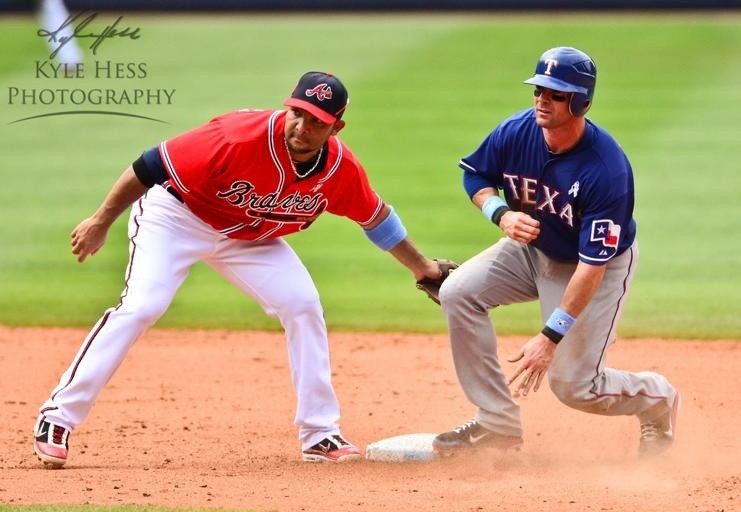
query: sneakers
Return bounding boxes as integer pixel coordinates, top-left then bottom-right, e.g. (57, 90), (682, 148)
(432, 418), (524, 456)
(33, 414), (70, 468)
(633, 389), (682, 466)
(302, 433), (363, 465)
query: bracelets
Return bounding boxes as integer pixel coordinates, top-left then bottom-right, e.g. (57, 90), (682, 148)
(542, 308), (576, 344)
(481, 195), (509, 224)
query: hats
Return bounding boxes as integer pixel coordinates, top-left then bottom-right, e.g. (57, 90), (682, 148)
(283, 70), (350, 126)
(522, 46), (597, 118)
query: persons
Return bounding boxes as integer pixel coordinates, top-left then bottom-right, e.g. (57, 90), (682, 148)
(433, 46), (679, 461)
(34, 71), (459, 464)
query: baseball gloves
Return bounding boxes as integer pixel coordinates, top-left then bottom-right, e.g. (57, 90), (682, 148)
(419, 259), (460, 303)
